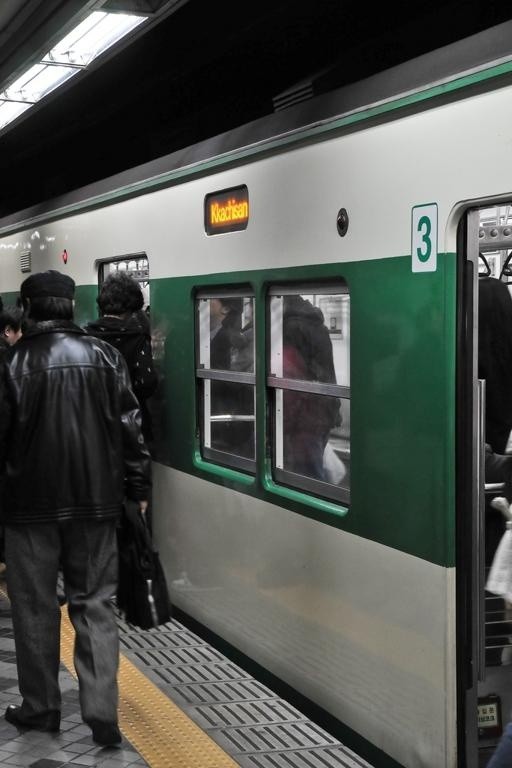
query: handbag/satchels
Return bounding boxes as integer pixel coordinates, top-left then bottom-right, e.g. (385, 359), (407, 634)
(117, 513), (170, 629)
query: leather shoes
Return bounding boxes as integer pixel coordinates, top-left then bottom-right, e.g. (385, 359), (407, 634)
(93, 725), (121, 744)
(6, 704), (61, 731)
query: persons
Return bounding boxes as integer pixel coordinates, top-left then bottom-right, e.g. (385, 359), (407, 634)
(0, 270), (152, 743)
(282, 294), (343, 483)
(2, 305), (22, 360)
(209, 297), (255, 460)
(81, 270), (155, 446)
(483, 443), (512, 666)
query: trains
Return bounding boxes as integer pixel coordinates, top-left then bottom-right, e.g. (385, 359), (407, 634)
(2, 15), (511, 768)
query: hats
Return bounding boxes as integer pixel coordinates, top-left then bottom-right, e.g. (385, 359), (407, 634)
(21, 270), (75, 298)
